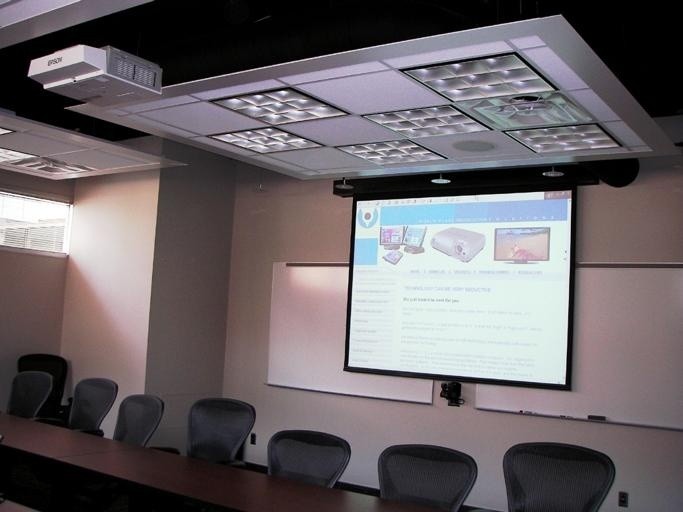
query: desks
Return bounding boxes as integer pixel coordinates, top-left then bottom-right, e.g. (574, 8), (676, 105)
(0, 410), (448, 512)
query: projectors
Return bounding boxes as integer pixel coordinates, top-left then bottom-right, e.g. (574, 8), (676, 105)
(28, 44), (163, 108)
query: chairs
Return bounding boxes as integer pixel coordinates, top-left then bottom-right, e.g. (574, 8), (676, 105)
(142, 394), (258, 471)
(264, 427), (352, 491)
(500, 440), (618, 511)
(14, 351), (69, 408)
(6, 370), (54, 424)
(30, 375), (118, 440)
(108, 391), (166, 447)
(375, 441), (479, 512)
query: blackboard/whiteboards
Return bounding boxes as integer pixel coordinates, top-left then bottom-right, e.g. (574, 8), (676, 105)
(263, 260), (434, 406)
(473, 261), (683, 432)
